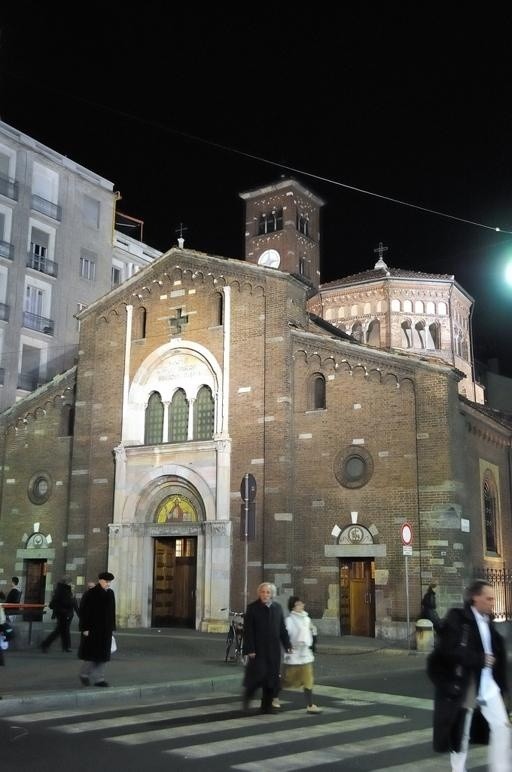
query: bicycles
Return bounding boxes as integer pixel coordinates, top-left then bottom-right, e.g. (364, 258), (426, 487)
(217, 606), (249, 665)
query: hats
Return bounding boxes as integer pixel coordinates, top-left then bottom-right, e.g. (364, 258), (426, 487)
(98, 572), (114, 581)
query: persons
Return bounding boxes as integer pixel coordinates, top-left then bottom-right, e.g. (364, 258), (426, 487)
(5, 577), (22, 626)
(0, 591), (11, 666)
(272, 595), (323, 713)
(66, 583), (80, 648)
(421, 582), (440, 632)
(79, 572), (116, 688)
(432, 579), (511, 771)
(38, 575), (75, 653)
(239, 582), (292, 715)
(77, 581), (96, 660)
(165, 499), (183, 522)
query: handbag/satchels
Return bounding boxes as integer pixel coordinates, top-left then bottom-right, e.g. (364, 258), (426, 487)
(111, 637), (118, 653)
(426, 647), (468, 699)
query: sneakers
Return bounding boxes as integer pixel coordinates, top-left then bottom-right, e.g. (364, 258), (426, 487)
(80, 676), (108, 687)
(262, 697), (323, 715)
